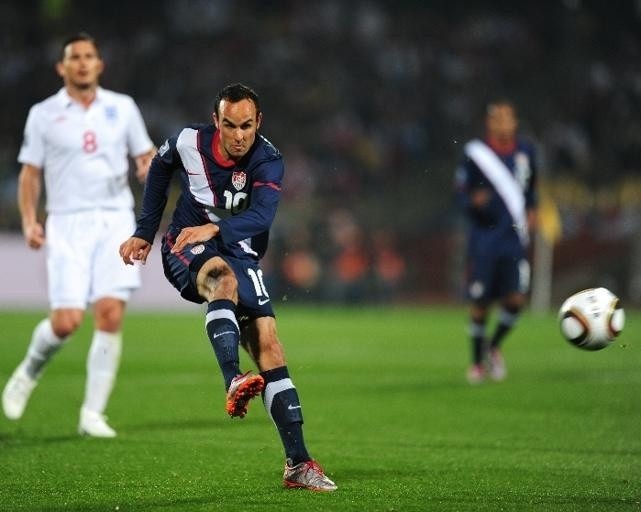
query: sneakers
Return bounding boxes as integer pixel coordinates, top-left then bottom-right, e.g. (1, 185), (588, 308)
(1, 359), (39, 422)
(281, 455), (339, 493)
(78, 403), (117, 439)
(462, 346), (506, 387)
(223, 369), (266, 422)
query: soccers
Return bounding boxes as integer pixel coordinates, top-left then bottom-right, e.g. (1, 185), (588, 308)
(558, 285), (627, 351)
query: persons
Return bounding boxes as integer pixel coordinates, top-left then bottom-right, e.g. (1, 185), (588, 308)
(450, 95), (540, 387)
(0, 32), (161, 440)
(117, 83), (341, 492)
